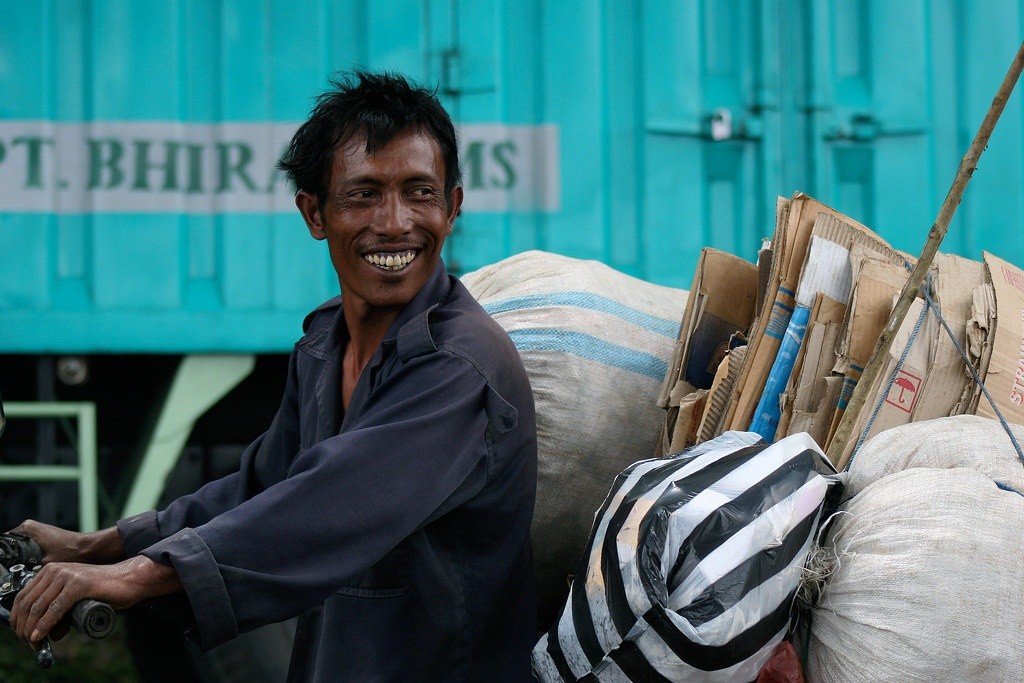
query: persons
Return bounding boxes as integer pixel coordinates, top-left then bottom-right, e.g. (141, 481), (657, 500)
(5, 68), (540, 683)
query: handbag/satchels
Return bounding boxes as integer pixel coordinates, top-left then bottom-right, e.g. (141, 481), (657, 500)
(527, 429), (842, 683)
